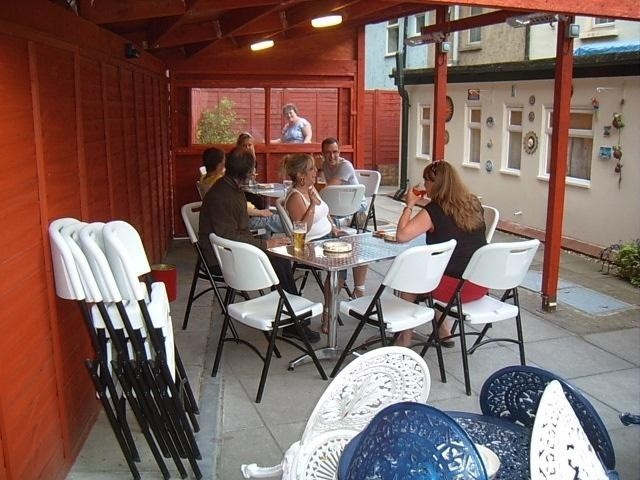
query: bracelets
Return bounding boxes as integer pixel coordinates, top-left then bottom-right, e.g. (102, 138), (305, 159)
(307, 208), (315, 215)
(330, 223), (337, 228)
(403, 205), (413, 213)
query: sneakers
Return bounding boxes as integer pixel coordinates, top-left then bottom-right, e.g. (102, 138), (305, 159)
(349, 289), (366, 301)
(282, 324), (321, 344)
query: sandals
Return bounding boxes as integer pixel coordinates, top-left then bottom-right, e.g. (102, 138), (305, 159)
(322, 308), (330, 334)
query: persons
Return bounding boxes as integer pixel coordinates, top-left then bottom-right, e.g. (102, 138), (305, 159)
(283, 154), (367, 333)
(391, 160), (488, 349)
(224, 130), (266, 207)
(308, 138), (368, 229)
(196, 147), (319, 343)
(263, 102), (313, 144)
(196, 146), (290, 234)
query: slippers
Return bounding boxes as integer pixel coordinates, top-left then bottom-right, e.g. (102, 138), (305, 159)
(432, 335), (455, 349)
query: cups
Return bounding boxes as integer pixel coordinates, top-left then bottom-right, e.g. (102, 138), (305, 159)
(316, 176), (326, 190)
(292, 219), (307, 250)
(282, 175), (294, 196)
(412, 182), (426, 196)
(248, 177), (257, 187)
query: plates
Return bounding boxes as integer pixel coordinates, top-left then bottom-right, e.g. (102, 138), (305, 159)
(322, 241), (353, 253)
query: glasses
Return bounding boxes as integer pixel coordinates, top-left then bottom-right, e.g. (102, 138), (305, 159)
(430, 161), (439, 176)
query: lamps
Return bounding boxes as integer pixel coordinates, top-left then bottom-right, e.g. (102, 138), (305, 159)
(310, 14), (343, 29)
(250, 40), (274, 52)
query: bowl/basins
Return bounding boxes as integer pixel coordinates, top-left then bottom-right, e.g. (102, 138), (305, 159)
(433, 441), (501, 480)
